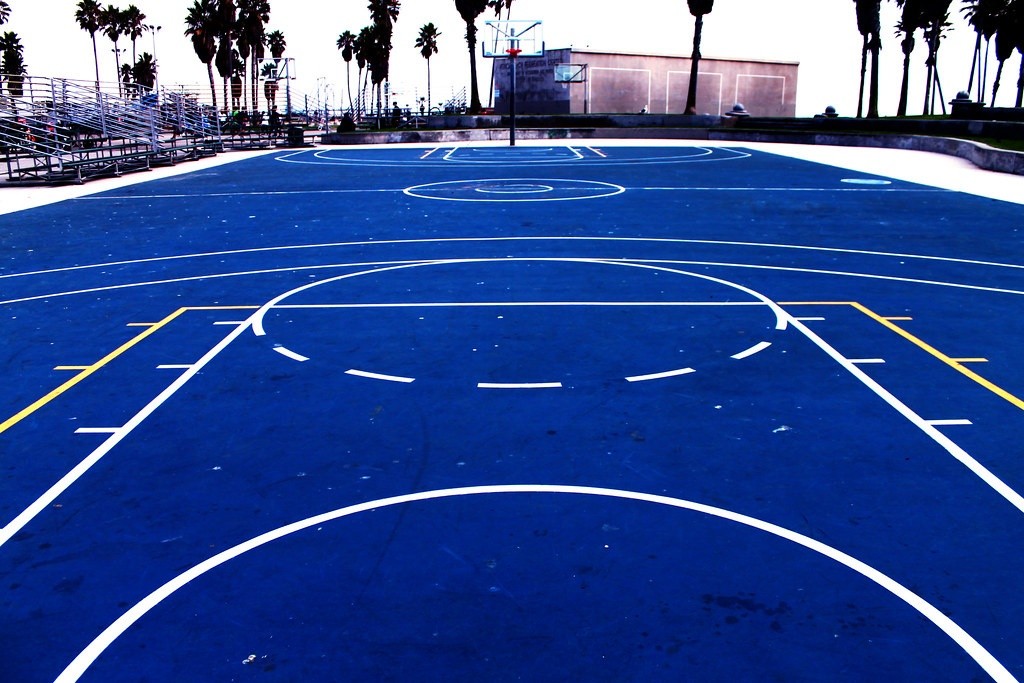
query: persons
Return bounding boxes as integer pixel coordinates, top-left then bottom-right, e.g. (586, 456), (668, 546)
(205, 106), (282, 137)
(392, 101), (400, 125)
